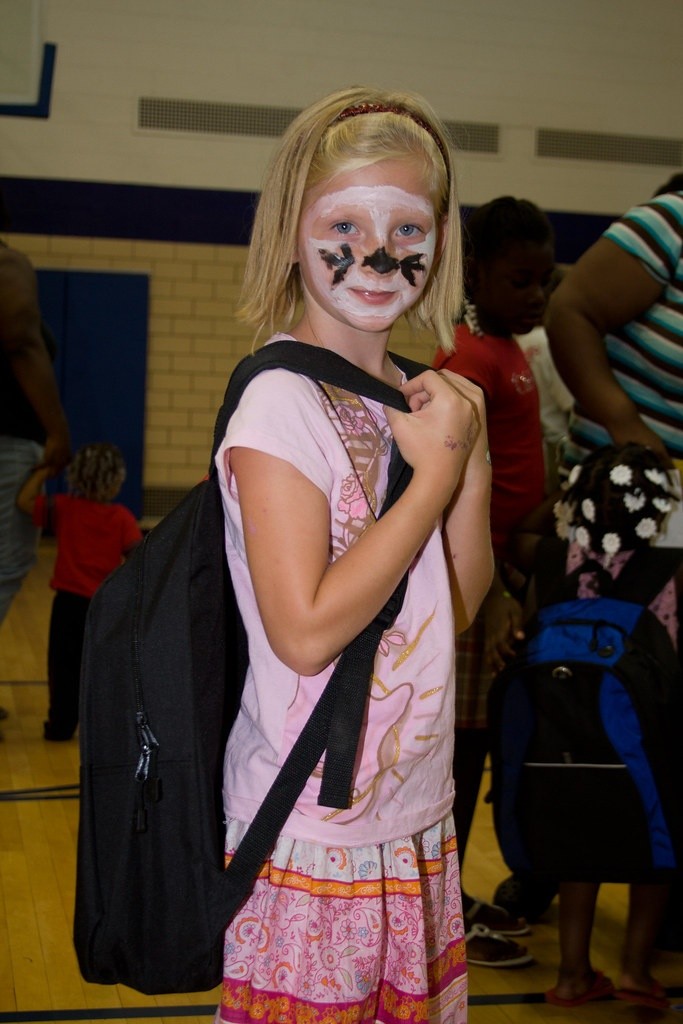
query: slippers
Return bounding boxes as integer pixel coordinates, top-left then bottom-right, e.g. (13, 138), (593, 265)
(465, 901), (530, 935)
(464, 923), (533, 966)
(545, 969), (614, 1007)
(614, 982), (669, 1008)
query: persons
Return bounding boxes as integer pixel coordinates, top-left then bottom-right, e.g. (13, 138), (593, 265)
(434, 161), (683, 1009)
(213, 86), (496, 1024)
(16, 431), (143, 744)
(0, 240), (76, 743)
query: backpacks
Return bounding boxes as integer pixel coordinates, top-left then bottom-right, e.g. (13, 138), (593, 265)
(484, 547), (683, 884)
(72, 340), (438, 995)
